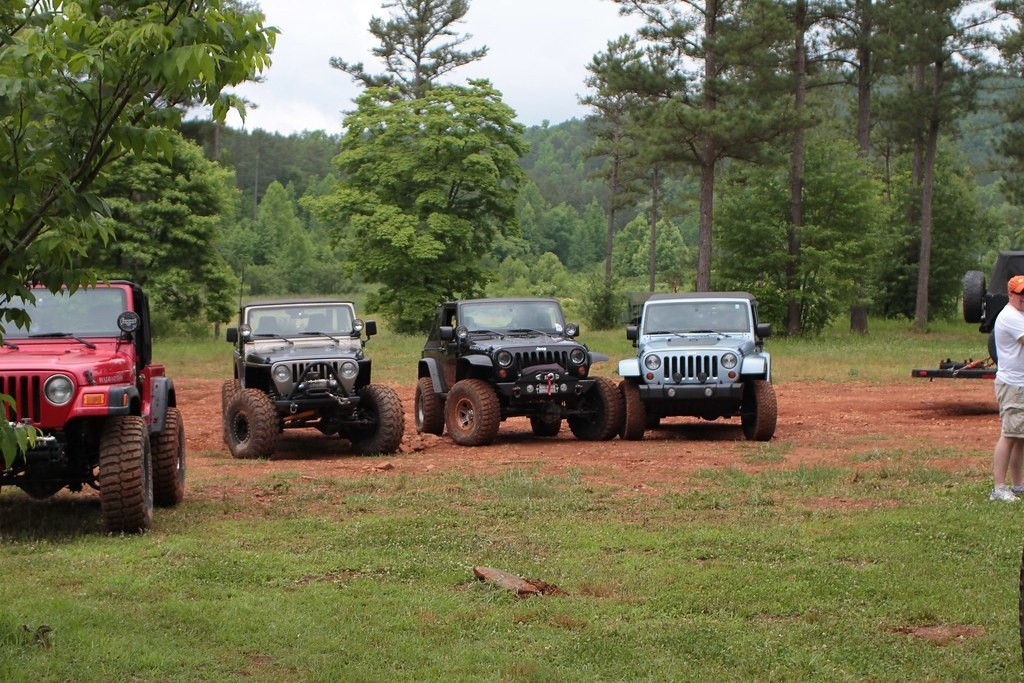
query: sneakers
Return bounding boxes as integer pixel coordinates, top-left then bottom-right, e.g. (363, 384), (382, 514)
(990, 483), (1024, 503)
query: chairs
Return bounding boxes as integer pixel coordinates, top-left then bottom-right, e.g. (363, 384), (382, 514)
(304, 313), (329, 331)
(253, 316), (282, 334)
(79, 302), (120, 331)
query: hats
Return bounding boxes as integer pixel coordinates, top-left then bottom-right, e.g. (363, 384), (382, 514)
(1008, 276), (1024, 296)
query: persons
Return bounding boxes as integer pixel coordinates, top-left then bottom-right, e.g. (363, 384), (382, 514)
(990, 276), (1024, 502)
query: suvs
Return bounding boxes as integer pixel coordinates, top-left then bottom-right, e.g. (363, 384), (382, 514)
(962, 251), (1024, 367)
(619, 290), (778, 442)
(221, 299), (406, 460)
(415, 298), (624, 446)
(0, 280), (185, 533)
